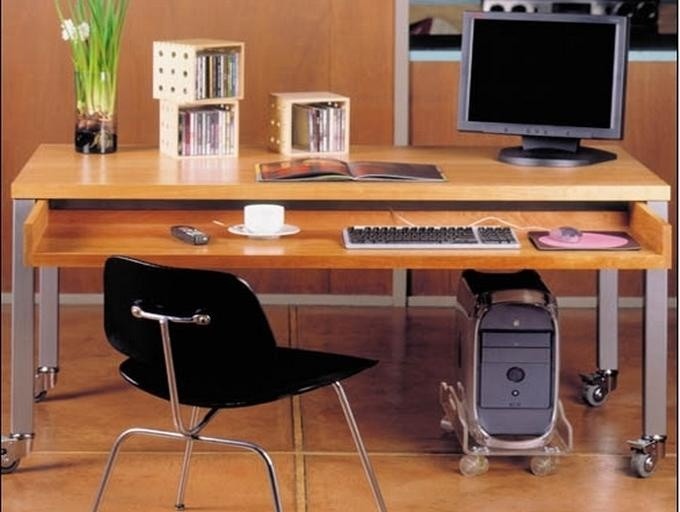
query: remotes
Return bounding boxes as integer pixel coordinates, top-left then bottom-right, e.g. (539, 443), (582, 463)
(171, 225), (210, 245)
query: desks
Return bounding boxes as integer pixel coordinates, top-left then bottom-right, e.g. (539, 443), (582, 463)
(1, 141), (673, 482)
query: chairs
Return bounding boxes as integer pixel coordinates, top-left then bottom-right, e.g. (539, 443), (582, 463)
(88, 253), (387, 512)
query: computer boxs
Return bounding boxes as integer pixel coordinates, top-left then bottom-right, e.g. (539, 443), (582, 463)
(451, 269), (560, 450)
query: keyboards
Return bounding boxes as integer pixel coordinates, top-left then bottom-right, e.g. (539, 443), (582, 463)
(343, 224), (522, 249)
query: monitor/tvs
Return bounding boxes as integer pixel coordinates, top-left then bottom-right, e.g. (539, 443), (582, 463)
(456, 9), (632, 167)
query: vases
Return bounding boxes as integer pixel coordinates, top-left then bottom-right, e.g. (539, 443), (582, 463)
(72, 69), (119, 154)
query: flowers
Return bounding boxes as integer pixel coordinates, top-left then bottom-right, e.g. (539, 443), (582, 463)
(56, 1), (128, 117)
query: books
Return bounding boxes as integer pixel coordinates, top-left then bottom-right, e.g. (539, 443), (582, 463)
(290, 103), (346, 154)
(253, 156), (449, 184)
(177, 51), (241, 156)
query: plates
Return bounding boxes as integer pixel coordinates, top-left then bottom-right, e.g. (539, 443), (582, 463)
(230, 223), (300, 239)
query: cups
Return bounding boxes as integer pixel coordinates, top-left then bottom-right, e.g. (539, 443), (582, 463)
(244, 204), (284, 232)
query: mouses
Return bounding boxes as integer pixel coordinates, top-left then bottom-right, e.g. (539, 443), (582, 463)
(549, 226), (581, 244)
